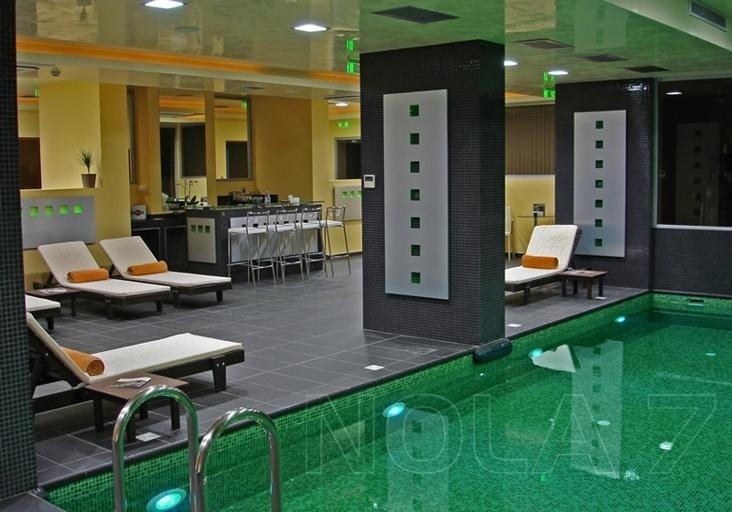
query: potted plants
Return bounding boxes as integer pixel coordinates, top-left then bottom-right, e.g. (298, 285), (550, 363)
(76, 145), (96, 188)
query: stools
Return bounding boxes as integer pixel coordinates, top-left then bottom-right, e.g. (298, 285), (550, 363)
(558, 270), (607, 298)
(25, 288), (79, 316)
(84, 370), (189, 442)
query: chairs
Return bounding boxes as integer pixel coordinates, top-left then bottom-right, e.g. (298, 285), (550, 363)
(227, 203), (352, 288)
(504, 225), (582, 304)
(26, 312), (245, 418)
(33, 241), (171, 320)
(25, 294), (60, 329)
(99, 236), (232, 309)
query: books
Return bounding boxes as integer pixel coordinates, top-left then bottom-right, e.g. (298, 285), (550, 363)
(108, 377), (151, 389)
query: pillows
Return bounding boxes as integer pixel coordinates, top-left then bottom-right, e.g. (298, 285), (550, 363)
(521, 254), (558, 269)
(67, 268), (108, 283)
(128, 260), (167, 275)
(63, 348), (104, 376)
